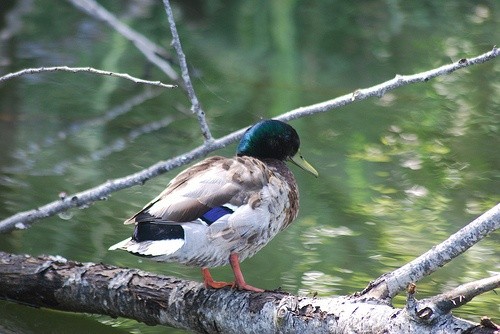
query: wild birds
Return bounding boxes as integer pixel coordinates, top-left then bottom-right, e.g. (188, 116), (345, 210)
(108, 119), (318, 293)
